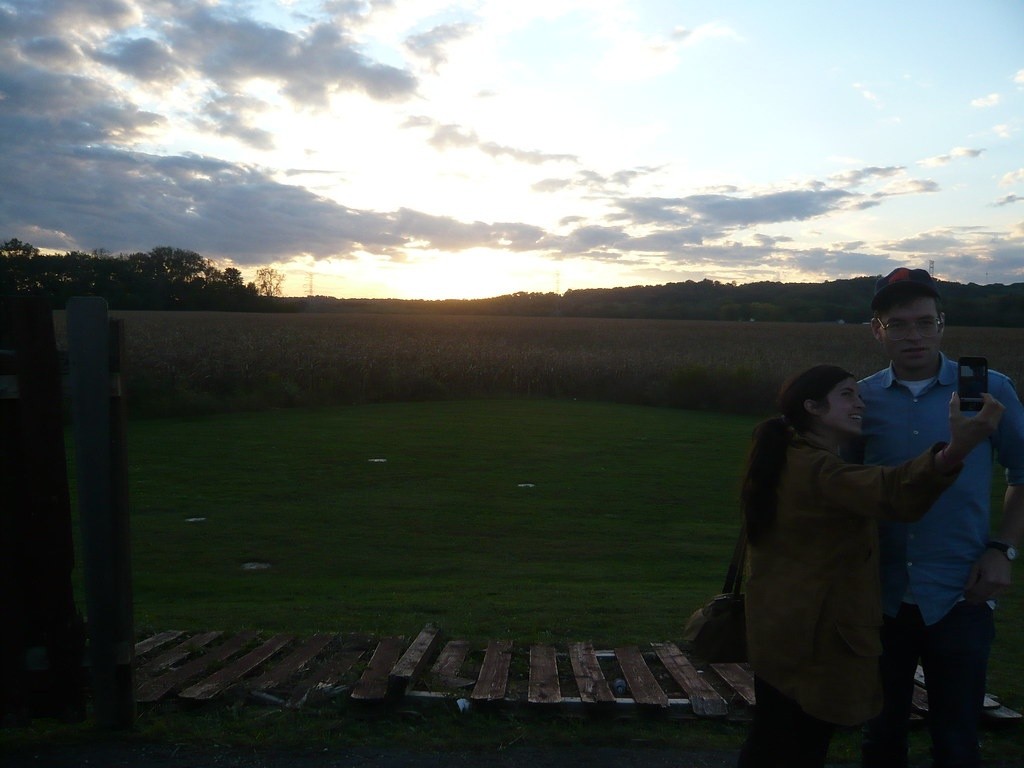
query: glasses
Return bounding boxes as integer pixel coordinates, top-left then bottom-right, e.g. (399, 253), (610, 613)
(877, 315), (943, 341)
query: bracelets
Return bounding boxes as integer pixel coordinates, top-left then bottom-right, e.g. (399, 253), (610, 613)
(942, 444), (950, 472)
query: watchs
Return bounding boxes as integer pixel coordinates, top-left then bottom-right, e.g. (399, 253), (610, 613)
(987, 540), (1017, 562)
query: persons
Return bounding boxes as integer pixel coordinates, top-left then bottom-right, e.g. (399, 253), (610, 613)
(851, 265), (1024, 767)
(737, 364), (1005, 767)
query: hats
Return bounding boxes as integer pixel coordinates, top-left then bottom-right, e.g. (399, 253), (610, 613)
(870, 266), (939, 312)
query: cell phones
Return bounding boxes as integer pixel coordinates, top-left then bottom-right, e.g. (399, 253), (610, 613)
(957, 356), (988, 412)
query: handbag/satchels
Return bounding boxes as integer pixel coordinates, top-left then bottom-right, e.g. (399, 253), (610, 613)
(684, 525), (751, 665)
